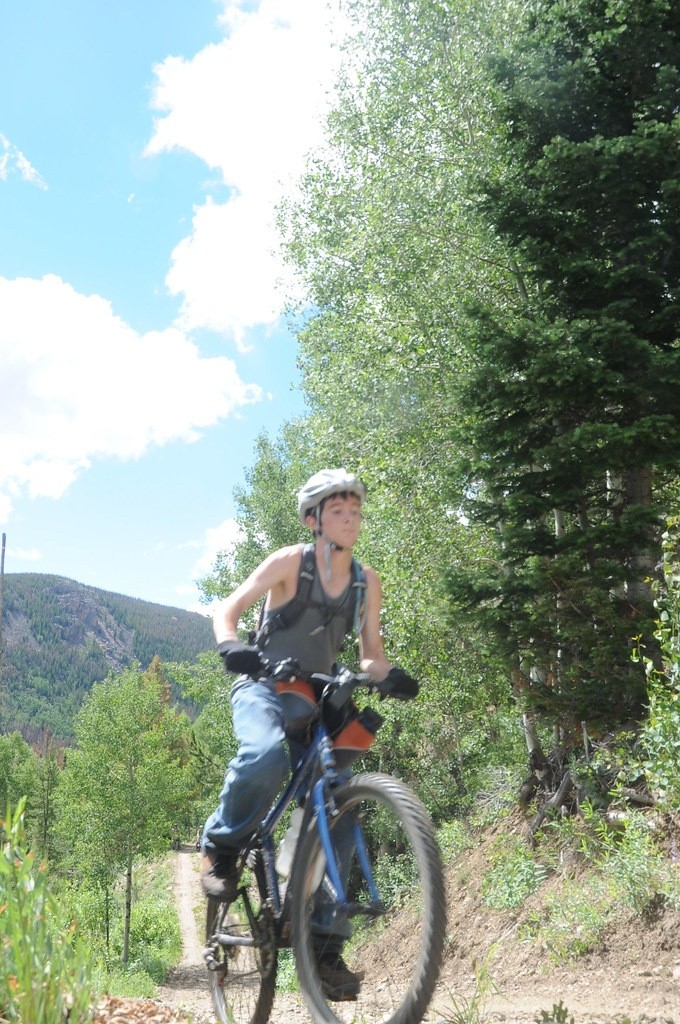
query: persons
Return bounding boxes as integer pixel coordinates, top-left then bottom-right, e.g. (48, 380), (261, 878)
(173, 824), (181, 847)
(196, 825), (204, 846)
(199, 467), (414, 1002)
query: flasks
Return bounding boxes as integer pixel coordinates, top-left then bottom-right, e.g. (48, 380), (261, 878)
(333, 707), (384, 752)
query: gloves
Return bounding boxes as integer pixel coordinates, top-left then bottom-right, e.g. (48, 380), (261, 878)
(376, 668), (419, 701)
(215, 641), (260, 675)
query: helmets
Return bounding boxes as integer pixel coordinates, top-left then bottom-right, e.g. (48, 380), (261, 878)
(297, 468), (366, 524)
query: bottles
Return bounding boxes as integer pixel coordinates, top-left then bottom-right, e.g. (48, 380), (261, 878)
(275, 797), (308, 878)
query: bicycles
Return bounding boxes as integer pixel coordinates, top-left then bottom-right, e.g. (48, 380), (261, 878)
(206, 651), (449, 1023)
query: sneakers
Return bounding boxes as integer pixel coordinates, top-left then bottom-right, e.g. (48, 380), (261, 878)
(314, 943), (362, 1003)
(199, 848), (239, 902)
